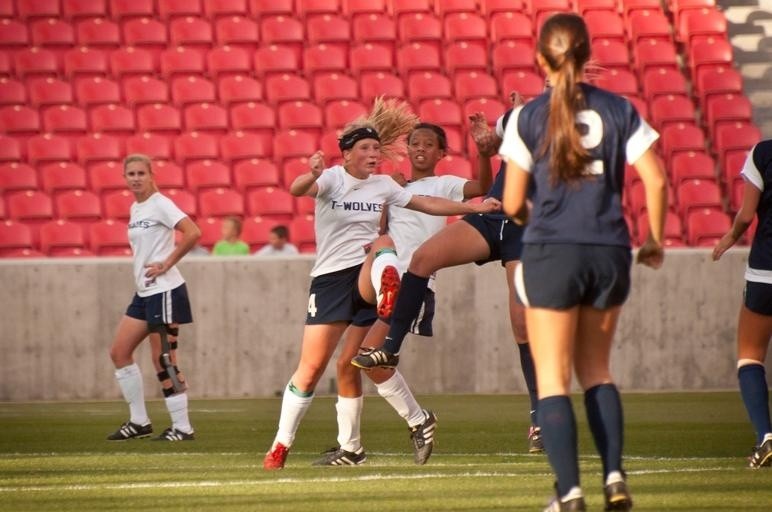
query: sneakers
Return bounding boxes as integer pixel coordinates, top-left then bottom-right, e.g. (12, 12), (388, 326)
(603, 482), (633, 512)
(377, 265), (401, 321)
(528, 426), (545, 453)
(350, 347), (400, 371)
(148, 427), (195, 442)
(310, 445), (368, 467)
(263, 439), (292, 470)
(408, 409), (438, 465)
(746, 440), (771, 468)
(106, 421), (152, 442)
(542, 495), (586, 512)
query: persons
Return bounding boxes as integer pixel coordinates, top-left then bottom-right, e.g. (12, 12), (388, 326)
(348, 56), (603, 458)
(174, 215), (209, 256)
(312, 108), (496, 467)
(250, 225), (300, 257)
(715, 139), (772, 469)
(263, 93), (504, 472)
(101, 153), (203, 444)
(496, 12), (670, 512)
(210, 218), (250, 257)
